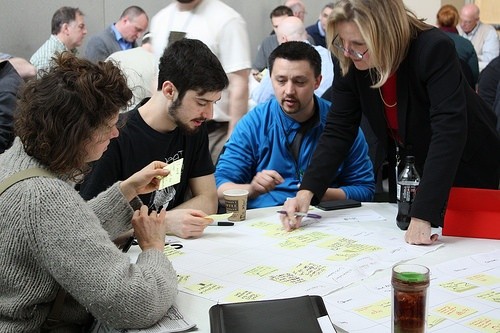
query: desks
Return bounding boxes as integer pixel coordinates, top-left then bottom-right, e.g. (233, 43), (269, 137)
(127, 202), (500, 333)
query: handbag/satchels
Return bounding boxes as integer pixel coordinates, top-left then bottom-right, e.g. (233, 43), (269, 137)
(209, 295), (337, 333)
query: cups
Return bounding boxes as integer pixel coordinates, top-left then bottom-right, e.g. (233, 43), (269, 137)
(391, 264), (431, 333)
(223, 189), (249, 222)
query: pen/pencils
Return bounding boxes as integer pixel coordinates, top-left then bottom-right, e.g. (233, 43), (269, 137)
(208, 221), (235, 226)
(277, 210), (322, 219)
(121, 203), (155, 256)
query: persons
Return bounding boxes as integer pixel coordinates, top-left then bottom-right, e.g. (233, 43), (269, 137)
(0, 0), (500, 333)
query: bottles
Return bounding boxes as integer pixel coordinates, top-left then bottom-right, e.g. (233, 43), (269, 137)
(396, 155), (421, 230)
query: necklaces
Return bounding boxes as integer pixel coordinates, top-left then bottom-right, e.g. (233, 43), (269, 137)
(377, 71), (397, 107)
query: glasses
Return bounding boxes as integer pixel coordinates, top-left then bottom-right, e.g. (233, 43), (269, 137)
(332, 34), (369, 59)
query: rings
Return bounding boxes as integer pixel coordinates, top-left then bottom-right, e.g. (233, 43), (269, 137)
(152, 212), (157, 217)
